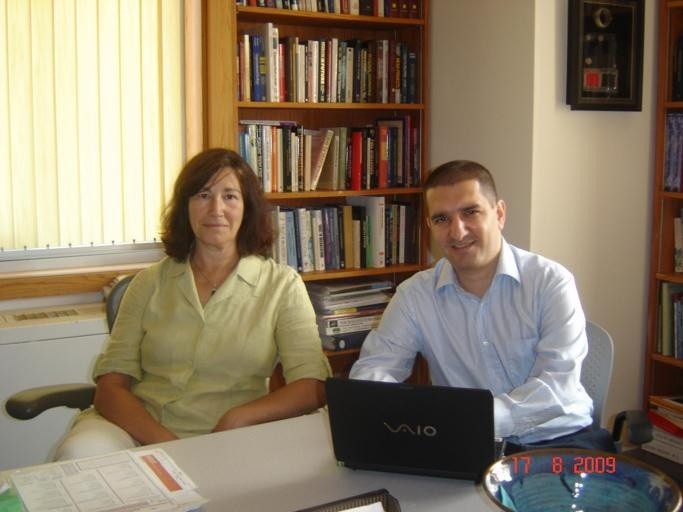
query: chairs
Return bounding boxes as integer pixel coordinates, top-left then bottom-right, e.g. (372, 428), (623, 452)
(579, 321), (615, 452)
(5, 275), (137, 421)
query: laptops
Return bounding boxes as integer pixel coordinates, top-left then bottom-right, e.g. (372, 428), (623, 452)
(324, 377), (494, 480)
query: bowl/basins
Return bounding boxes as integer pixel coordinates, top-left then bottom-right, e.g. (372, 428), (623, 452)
(480, 447), (681, 512)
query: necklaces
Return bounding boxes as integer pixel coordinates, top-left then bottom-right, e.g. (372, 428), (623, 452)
(193, 257), (240, 296)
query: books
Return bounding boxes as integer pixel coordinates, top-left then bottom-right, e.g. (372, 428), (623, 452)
(237, 23), (417, 105)
(665, 110), (683, 191)
(672, 37), (683, 101)
(236, 0), (420, 19)
(270, 196), (418, 273)
(656, 281), (683, 360)
(673, 217), (683, 272)
(307, 279), (394, 352)
(640, 394), (683, 465)
(239, 114), (421, 193)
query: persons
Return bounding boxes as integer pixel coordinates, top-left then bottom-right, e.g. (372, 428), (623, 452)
(53, 148), (332, 462)
(348, 159), (617, 458)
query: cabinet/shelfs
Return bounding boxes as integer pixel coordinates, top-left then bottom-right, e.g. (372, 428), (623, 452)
(202, 0), (430, 393)
(646, 1), (682, 486)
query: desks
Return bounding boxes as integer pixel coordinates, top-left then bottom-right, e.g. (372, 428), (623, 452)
(0, 410), (530, 512)
